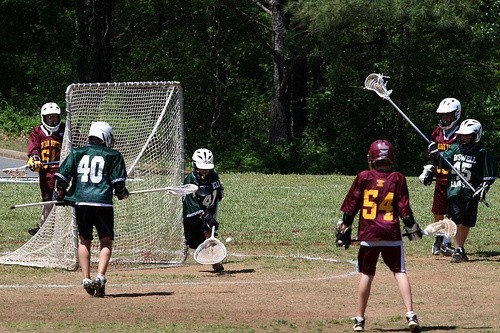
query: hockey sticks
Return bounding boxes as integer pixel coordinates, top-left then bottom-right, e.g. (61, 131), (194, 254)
(337, 217), (458, 246)
(2, 161), (60, 177)
(11, 184), (199, 209)
(193, 199), (227, 265)
(364, 73), (490, 207)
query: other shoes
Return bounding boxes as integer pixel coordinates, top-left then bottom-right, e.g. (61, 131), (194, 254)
(431, 234), (453, 254)
(28, 223), (45, 238)
(92, 274), (107, 298)
(82, 277), (96, 295)
(352, 313), (365, 330)
(212, 263), (225, 272)
(406, 311), (419, 330)
(452, 246), (467, 261)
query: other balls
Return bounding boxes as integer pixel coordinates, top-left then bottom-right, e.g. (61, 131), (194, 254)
(226, 237), (232, 242)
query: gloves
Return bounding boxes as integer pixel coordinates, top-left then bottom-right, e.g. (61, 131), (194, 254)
(403, 223), (425, 241)
(54, 187), (64, 201)
(115, 185), (129, 201)
(418, 164), (435, 187)
(27, 155), (40, 170)
(333, 222), (352, 250)
(472, 181), (491, 201)
(426, 141), (442, 164)
(202, 212), (221, 230)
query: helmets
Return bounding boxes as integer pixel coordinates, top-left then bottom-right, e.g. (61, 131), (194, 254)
(88, 118), (112, 149)
(368, 137), (393, 163)
(191, 147), (216, 172)
(456, 119), (482, 143)
(435, 97), (461, 130)
(40, 102), (62, 131)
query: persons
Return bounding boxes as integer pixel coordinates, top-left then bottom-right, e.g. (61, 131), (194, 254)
(428, 119), (496, 263)
(419, 98), (462, 257)
(335, 140), (423, 333)
(27, 102), (82, 235)
(52, 122), (128, 297)
(183, 148), (224, 273)
